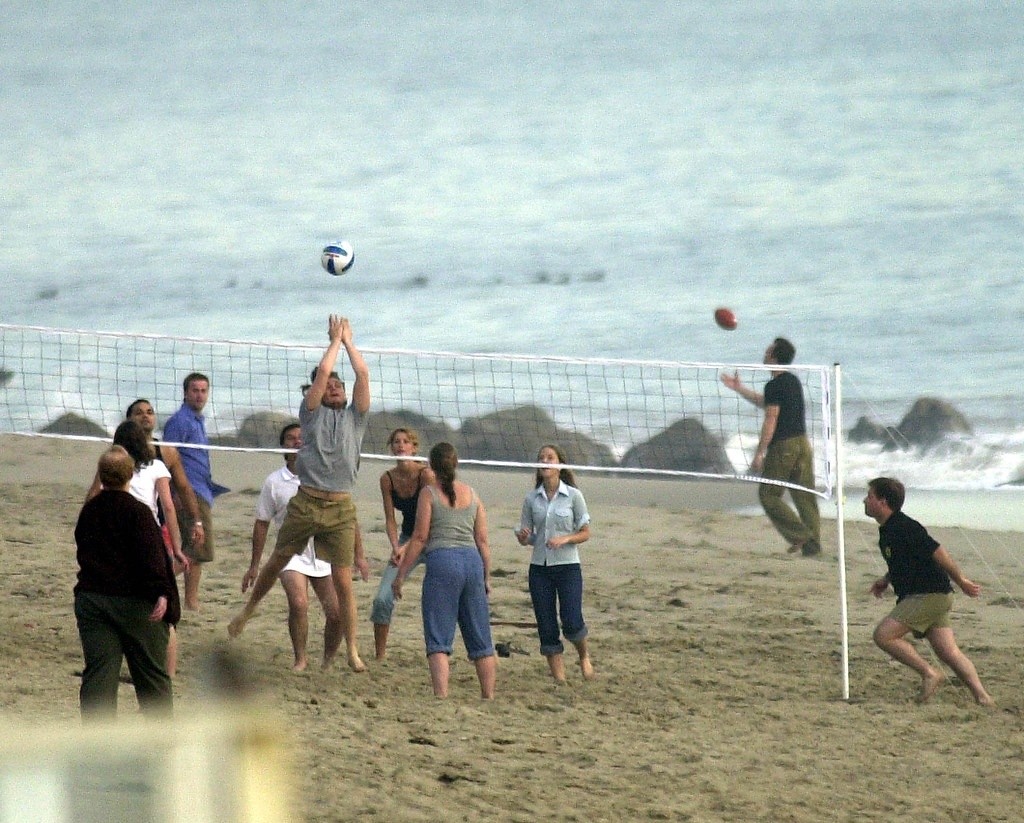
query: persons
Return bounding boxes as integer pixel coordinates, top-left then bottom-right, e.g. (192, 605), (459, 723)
(863, 478), (992, 704)
(228, 313), (370, 673)
(243, 425), (369, 675)
(720, 338), (821, 556)
(370, 427), (497, 699)
(514, 445), (592, 680)
(74, 373), (230, 720)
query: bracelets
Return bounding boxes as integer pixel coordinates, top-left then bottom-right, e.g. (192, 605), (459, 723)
(195, 522), (203, 526)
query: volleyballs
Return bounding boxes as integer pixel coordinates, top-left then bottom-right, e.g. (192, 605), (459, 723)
(321, 239), (355, 276)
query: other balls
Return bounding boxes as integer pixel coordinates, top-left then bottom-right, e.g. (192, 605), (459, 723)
(714, 307), (739, 332)
